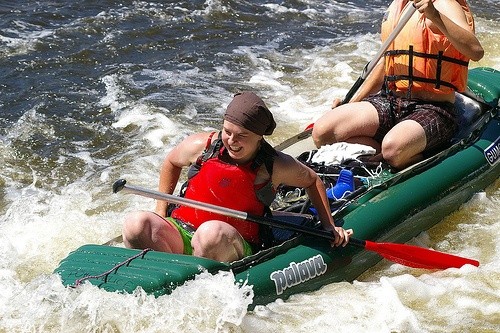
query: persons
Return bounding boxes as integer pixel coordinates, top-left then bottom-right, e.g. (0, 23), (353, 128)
(121, 91), (354, 265)
(311, 0), (485, 172)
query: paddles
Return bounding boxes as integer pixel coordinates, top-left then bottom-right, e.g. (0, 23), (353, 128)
(110, 179), (481, 271)
(305, 2), (417, 129)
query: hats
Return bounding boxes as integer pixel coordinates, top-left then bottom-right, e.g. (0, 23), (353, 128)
(224, 91), (277, 137)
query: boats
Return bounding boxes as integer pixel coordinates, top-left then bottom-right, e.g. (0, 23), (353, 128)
(53, 67), (500, 311)
